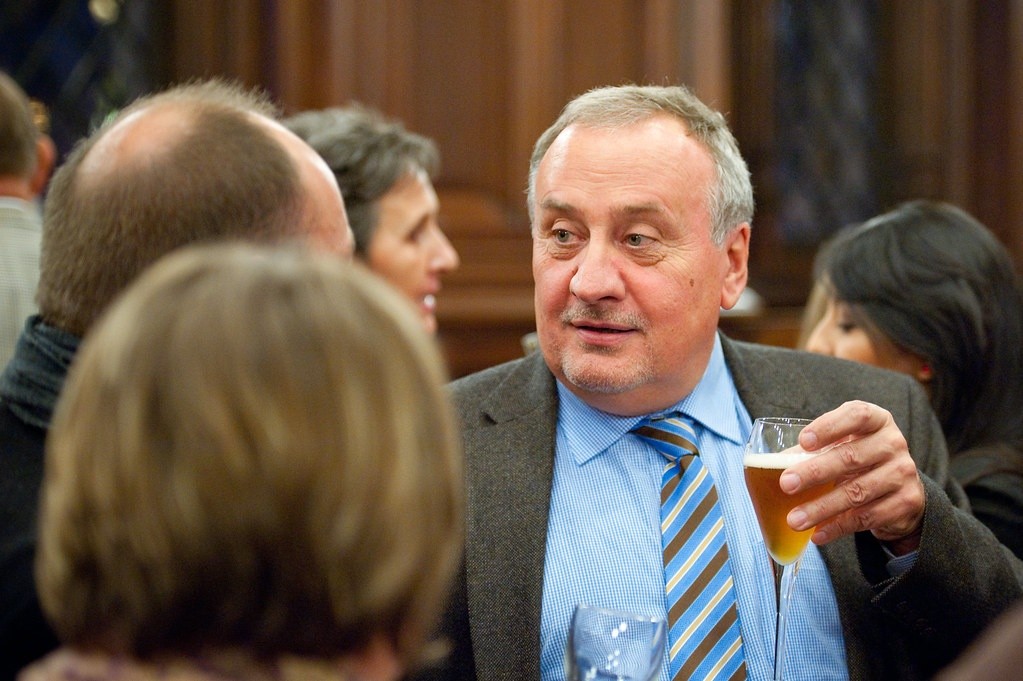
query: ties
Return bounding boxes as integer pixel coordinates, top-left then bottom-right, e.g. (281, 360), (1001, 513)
(625, 413), (747, 680)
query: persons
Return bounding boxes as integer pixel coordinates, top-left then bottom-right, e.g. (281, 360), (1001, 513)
(419, 84), (1023, 679)
(2, 249), (458, 677)
(0, 0), (165, 162)
(791, 198), (1023, 552)
(0, 82), (361, 675)
(0, 74), (61, 386)
(278, 100), (462, 327)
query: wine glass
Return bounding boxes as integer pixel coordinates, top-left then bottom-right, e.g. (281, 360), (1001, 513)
(746, 411), (829, 681)
(565, 602), (669, 679)
